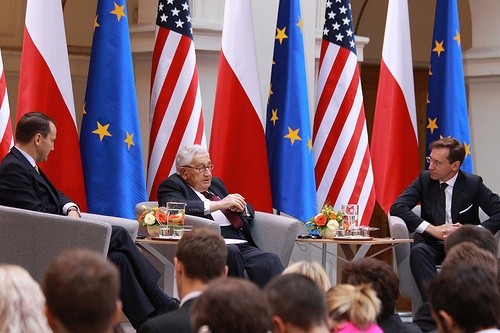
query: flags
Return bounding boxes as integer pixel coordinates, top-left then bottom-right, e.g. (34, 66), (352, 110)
(145, 0), (207, 201)
(313, 0), (378, 232)
(206, 1), (279, 213)
(14, 1), (88, 215)
(265, 1), (319, 236)
(370, 0), (419, 219)
(424, 0), (473, 172)
(80, 0), (148, 220)
(0, 47), (15, 162)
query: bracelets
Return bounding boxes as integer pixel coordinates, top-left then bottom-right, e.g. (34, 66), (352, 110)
(67, 207), (81, 217)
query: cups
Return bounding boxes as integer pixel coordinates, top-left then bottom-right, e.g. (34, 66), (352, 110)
(184, 225), (193, 238)
(337, 226), (346, 238)
(159, 224), (169, 237)
(360, 226), (370, 238)
(352, 227), (360, 238)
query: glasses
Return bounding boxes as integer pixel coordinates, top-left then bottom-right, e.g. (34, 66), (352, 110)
(425, 155), (451, 169)
(180, 163), (214, 174)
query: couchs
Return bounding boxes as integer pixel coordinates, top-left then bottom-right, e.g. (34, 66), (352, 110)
(134, 201), (300, 301)
(0, 205), (141, 289)
(388, 208), (500, 323)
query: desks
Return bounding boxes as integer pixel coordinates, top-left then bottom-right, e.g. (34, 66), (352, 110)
(134, 235), (248, 300)
(292, 236), (415, 314)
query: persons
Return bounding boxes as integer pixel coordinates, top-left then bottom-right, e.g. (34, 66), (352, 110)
(388, 137), (499, 300)
(156, 145), (288, 287)
(1, 113), (179, 329)
(1, 223), (499, 333)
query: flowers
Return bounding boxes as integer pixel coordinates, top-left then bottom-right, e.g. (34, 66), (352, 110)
(303, 205), (350, 239)
(136, 205), (185, 230)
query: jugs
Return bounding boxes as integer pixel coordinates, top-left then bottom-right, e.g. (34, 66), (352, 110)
(340, 204), (360, 238)
(167, 202), (187, 238)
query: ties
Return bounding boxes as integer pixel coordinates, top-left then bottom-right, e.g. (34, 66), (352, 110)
(200, 191), (244, 231)
(435, 183), (449, 241)
(35, 165), (40, 174)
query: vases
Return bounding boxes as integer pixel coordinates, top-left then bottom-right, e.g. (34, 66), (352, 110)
(321, 227), (336, 240)
(146, 225), (162, 237)
